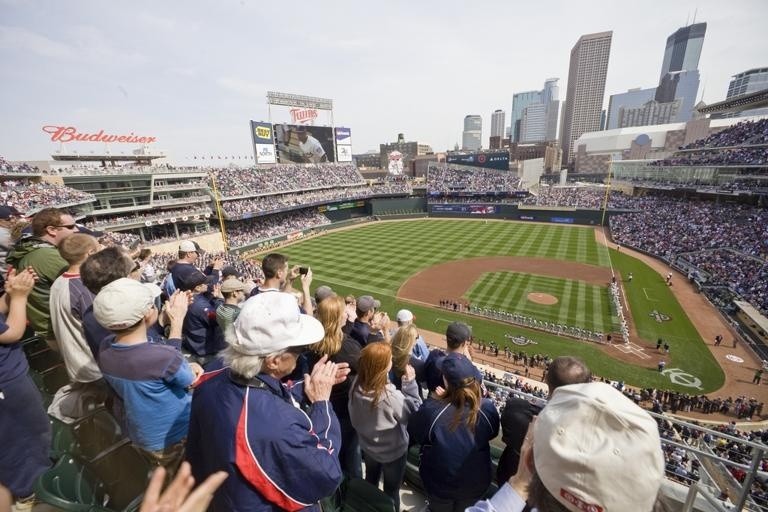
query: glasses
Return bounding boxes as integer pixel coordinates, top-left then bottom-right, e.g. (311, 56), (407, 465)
(129, 261), (141, 274)
(39, 224), (77, 230)
(284, 346), (307, 355)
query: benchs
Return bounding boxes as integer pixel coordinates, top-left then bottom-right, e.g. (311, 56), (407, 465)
(285, 128), (312, 161)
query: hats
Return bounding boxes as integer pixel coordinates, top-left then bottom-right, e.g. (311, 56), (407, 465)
(356, 295), (380, 312)
(229, 291), (325, 356)
(0, 205), (26, 220)
(178, 240), (206, 256)
(294, 126), (306, 133)
(93, 277), (163, 331)
(74, 225), (104, 237)
(396, 309), (414, 322)
(445, 320), (472, 343)
(436, 352), (476, 387)
(314, 286), (335, 300)
(533, 382), (665, 512)
(219, 266), (246, 293)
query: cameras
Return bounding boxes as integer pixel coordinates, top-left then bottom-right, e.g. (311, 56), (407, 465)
(298, 267), (308, 275)
(214, 257), (222, 261)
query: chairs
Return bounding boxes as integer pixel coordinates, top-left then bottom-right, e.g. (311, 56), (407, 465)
(320, 460), (429, 512)
(19, 322), (166, 512)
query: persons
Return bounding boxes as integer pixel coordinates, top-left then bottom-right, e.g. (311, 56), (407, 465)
(0, 118), (768, 512)
